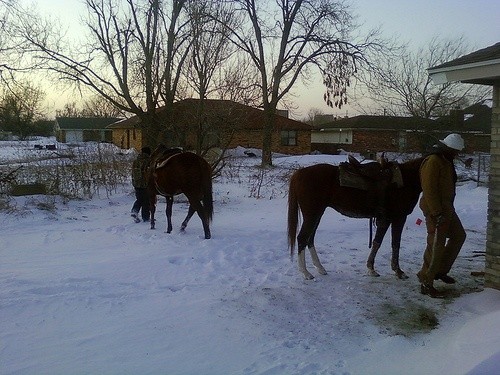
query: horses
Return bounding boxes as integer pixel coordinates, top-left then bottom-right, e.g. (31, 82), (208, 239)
(287, 152), (436, 280)
(143, 144), (214, 240)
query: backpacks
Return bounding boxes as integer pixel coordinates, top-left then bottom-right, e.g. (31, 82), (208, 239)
(131, 156), (151, 188)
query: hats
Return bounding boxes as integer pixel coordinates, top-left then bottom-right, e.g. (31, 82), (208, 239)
(439, 132), (465, 151)
(141, 146), (151, 154)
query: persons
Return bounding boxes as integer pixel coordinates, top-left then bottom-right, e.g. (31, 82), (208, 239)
(419, 134), (467, 298)
(131, 146), (156, 223)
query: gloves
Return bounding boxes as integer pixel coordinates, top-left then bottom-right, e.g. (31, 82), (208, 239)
(430, 212), (449, 228)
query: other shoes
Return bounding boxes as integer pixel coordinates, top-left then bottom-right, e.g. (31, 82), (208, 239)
(421, 283), (439, 298)
(434, 272), (456, 284)
(129, 208), (141, 223)
